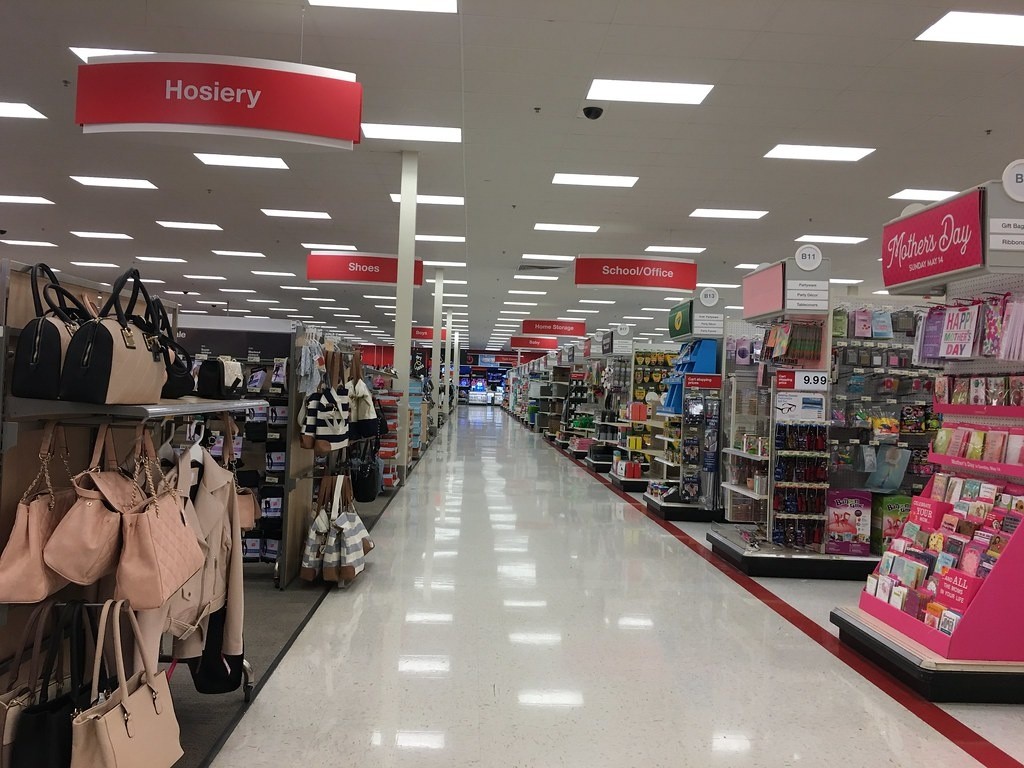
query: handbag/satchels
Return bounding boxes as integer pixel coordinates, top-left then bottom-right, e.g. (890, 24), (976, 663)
(198, 356), (244, 400)
(12, 263), (91, 400)
(299, 475), (374, 581)
(144, 298), (195, 398)
(345, 351), (379, 437)
(356, 439), (382, 502)
(374, 399), (388, 434)
(222, 412), (261, 532)
(0, 599), (185, 768)
(62, 268), (168, 405)
(297, 350), (351, 456)
(0, 421), (206, 611)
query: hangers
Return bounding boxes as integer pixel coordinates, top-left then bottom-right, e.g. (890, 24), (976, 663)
(118, 416), (234, 496)
(303, 326), (340, 354)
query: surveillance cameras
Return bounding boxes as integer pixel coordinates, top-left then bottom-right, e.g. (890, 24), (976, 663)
(0, 230), (7, 234)
(183, 291), (188, 294)
(583, 107), (603, 120)
(212, 305), (216, 307)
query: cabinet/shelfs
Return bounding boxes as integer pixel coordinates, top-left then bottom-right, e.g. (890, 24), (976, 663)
(528, 359), (548, 431)
(706, 244), (945, 581)
(543, 350), (570, 441)
(533, 354), (563, 433)
(422, 356), (528, 452)
(642, 286), (766, 524)
(313, 330), (398, 516)
(0, 260), (270, 767)
(164, 313), (365, 589)
(554, 345), (588, 450)
(566, 331), (607, 459)
(602, 323), (688, 492)
(830, 157), (1024, 706)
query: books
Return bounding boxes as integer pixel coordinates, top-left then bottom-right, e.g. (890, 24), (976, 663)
(866, 301), (1024, 637)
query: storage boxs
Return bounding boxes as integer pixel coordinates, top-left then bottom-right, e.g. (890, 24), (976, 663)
(612, 399), (647, 480)
(528, 366), (569, 435)
(372, 378), (422, 490)
